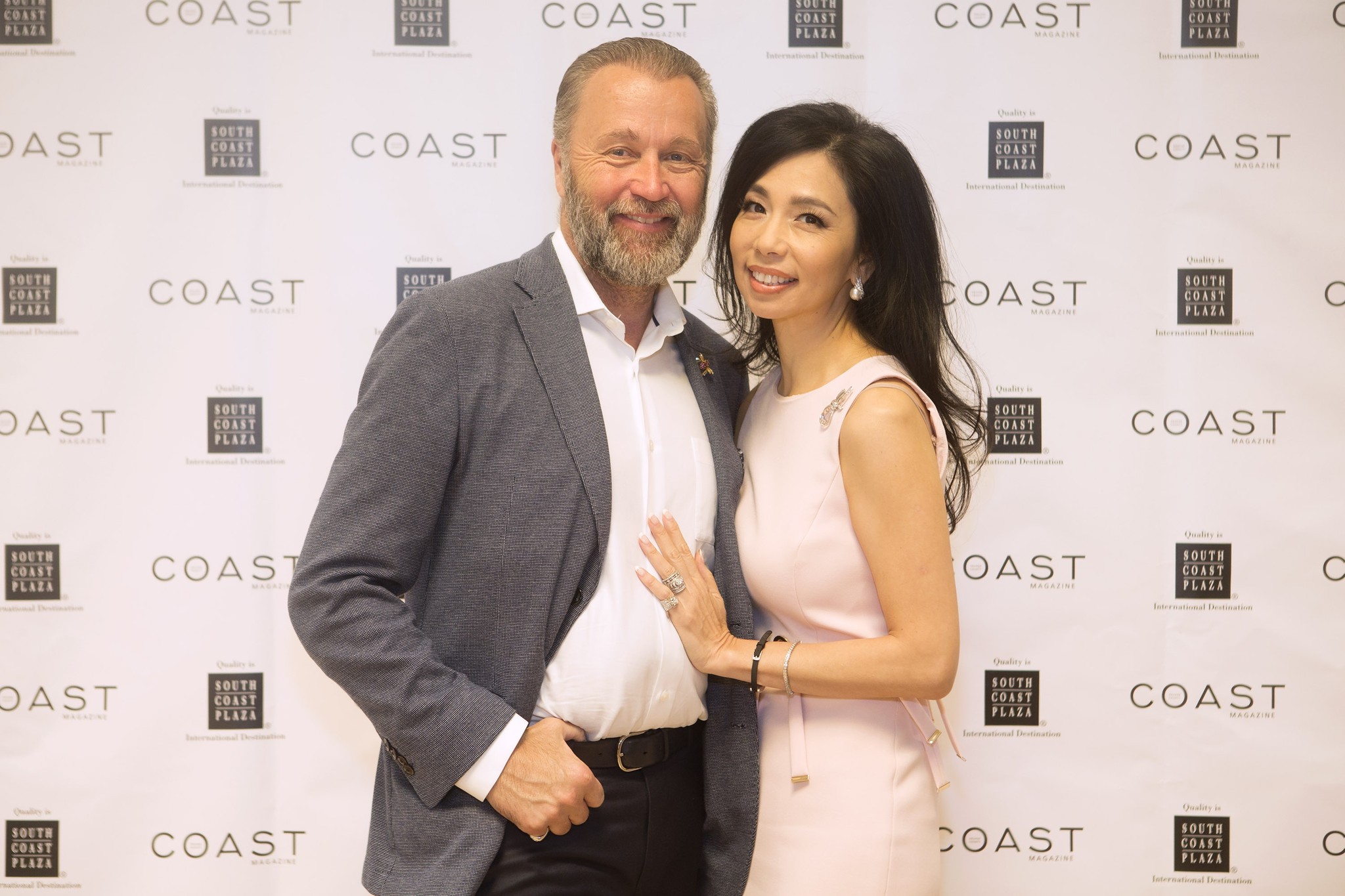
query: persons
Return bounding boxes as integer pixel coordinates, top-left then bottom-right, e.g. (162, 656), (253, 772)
(288, 37), (760, 896)
(638, 103), (992, 896)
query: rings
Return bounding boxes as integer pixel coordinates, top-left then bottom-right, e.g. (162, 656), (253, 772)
(530, 828), (549, 842)
(660, 597), (679, 611)
(662, 570), (687, 594)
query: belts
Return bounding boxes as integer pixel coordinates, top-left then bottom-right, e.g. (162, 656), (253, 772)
(564, 719), (707, 773)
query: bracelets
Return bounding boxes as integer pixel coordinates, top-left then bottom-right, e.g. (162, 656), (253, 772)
(751, 631), (789, 695)
(783, 638), (801, 696)
